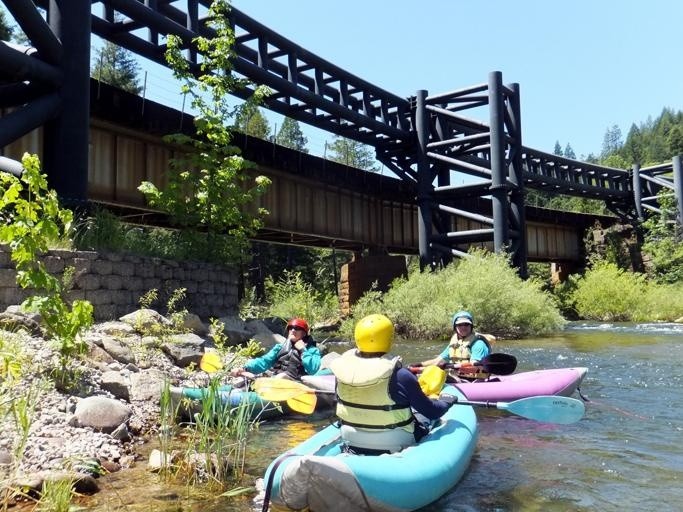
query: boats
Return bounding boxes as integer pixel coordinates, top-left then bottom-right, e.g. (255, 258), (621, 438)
(260, 383), (482, 512)
(164, 357), (402, 426)
(300, 364), (592, 404)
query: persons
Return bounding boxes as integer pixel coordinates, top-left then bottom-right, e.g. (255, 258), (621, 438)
(408, 311), (491, 383)
(231, 319), (321, 391)
(335, 313), (457, 455)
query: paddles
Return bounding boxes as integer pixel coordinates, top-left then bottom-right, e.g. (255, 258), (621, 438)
(412, 353), (518, 375)
(199, 353), (248, 384)
(455, 397), (586, 425)
(253, 378), (338, 414)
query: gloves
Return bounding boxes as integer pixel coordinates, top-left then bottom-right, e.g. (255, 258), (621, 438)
(438, 392), (458, 407)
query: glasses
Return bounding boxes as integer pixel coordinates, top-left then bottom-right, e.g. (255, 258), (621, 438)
(286, 326), (303, 331)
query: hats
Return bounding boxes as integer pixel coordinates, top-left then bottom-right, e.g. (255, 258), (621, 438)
(454, 317), (473, 325)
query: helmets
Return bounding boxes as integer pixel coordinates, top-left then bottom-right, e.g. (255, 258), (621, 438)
(354, 313), (396, 355)
(285, 318), (309, 334)
